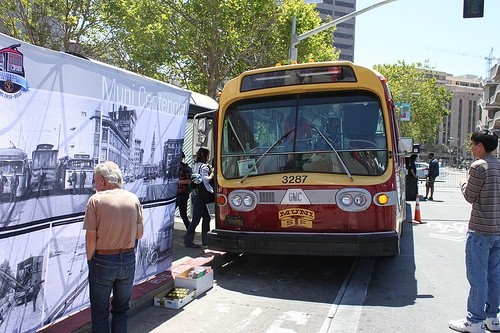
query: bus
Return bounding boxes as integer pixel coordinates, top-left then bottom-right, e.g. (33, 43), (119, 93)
(192, 60), (421, 258)
(59, 153), (98, 193)
(27, 144), (60, 195)
(0, 140), (31, 201)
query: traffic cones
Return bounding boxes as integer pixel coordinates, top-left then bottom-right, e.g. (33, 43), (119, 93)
(412, 195), (427, 224)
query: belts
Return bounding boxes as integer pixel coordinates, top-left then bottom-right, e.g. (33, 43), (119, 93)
(95, 248), (134, 255)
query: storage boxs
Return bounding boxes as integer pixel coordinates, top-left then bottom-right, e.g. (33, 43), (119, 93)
(175, 267), (213, 297)
(154, 286), (197, 310)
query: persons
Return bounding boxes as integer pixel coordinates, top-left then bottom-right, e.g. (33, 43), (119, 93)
(79, 167), (86, 194)
(9, 170), (19, 202)
(175, 151), (196, 237)
(283, 106), (313, 140)
(184, 148), (214, 248)
(33, 167), (46, 198)
(405, 154), (419, 199)
(68, 169), (77, 194)
(31, 279), (40, 312)
(83, 161), (144, 333)
(0, 171), (8, 203)
(423, 153), (440, 200)
(448, 129), (500, 333)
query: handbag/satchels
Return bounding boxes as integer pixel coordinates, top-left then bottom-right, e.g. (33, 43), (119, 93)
(196, 164), (216, 204)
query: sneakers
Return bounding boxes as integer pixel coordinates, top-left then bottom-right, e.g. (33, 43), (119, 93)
(482, 316), (500, 332)
(448, 317), (486, 333)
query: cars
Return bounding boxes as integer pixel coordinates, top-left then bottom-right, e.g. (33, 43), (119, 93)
(402, 162), (430, 179)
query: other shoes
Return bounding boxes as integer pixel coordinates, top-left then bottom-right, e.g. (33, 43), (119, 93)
(428, 197), (433, 201)
(186, 243), (201, 248)
(423, 197), (428, 199)
